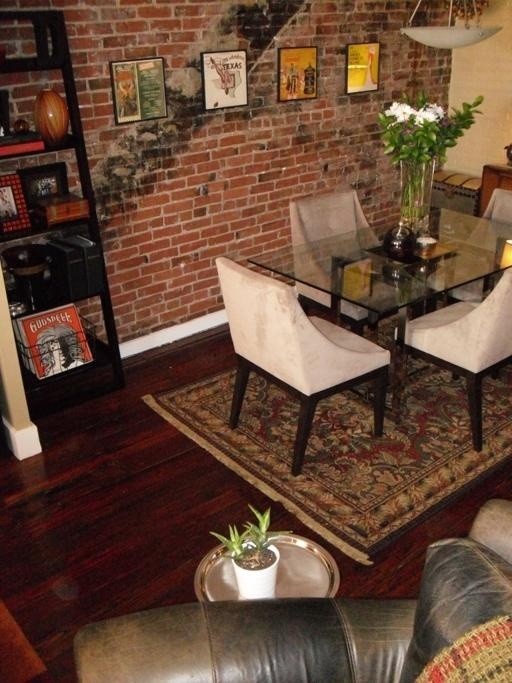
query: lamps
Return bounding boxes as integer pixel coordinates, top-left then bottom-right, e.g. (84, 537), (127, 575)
(499, 238), (512, 274)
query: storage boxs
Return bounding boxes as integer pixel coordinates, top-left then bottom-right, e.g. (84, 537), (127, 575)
(15, 312), (100, 390)
(32, 190), (92, 229)
(428, 166), (485, 218)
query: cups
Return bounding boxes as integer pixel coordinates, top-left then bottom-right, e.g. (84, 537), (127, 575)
(417, 237), (436, 255)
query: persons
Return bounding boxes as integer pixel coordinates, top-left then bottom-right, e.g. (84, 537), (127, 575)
(36, 325), (88, 378)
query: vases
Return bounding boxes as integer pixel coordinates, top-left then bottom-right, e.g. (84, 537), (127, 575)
(396, 157), (437, 239)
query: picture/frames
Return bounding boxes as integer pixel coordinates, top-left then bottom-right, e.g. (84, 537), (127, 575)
(342, 38), (382, 97)
(199, 48), (251, 112)
(109, 56), (170, 126)
(17, 160), (71, 231)
(275, 45), (320, 105)
(0, 171), (39, 244)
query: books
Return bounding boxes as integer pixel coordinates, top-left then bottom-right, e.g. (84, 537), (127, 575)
(12, 302), (94, 381)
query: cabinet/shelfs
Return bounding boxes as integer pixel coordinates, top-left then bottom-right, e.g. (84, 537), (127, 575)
(0, 7), (129, 425)
(476, 160), (512, 220)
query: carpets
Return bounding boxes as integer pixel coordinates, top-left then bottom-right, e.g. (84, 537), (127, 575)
(139, 292), (511, 571)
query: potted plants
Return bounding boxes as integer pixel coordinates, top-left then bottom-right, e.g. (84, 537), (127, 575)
(207, 502), (294, 602)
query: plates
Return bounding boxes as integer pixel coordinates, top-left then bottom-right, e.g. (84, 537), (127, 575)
(193, 534), (341, 602)
(415, 242), (460, 261)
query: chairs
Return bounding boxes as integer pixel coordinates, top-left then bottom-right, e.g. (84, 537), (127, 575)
(213, 254), (393, 477)
(287, 188), (401, 337)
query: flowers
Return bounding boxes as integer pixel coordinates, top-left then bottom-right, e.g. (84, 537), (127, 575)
(374, 87), (486, 221)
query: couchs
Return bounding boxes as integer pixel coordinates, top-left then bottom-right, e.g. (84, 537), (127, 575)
(424, 187), (512, 305)
(464, 495), (511, 569)
(70, 534), (511, 683)
